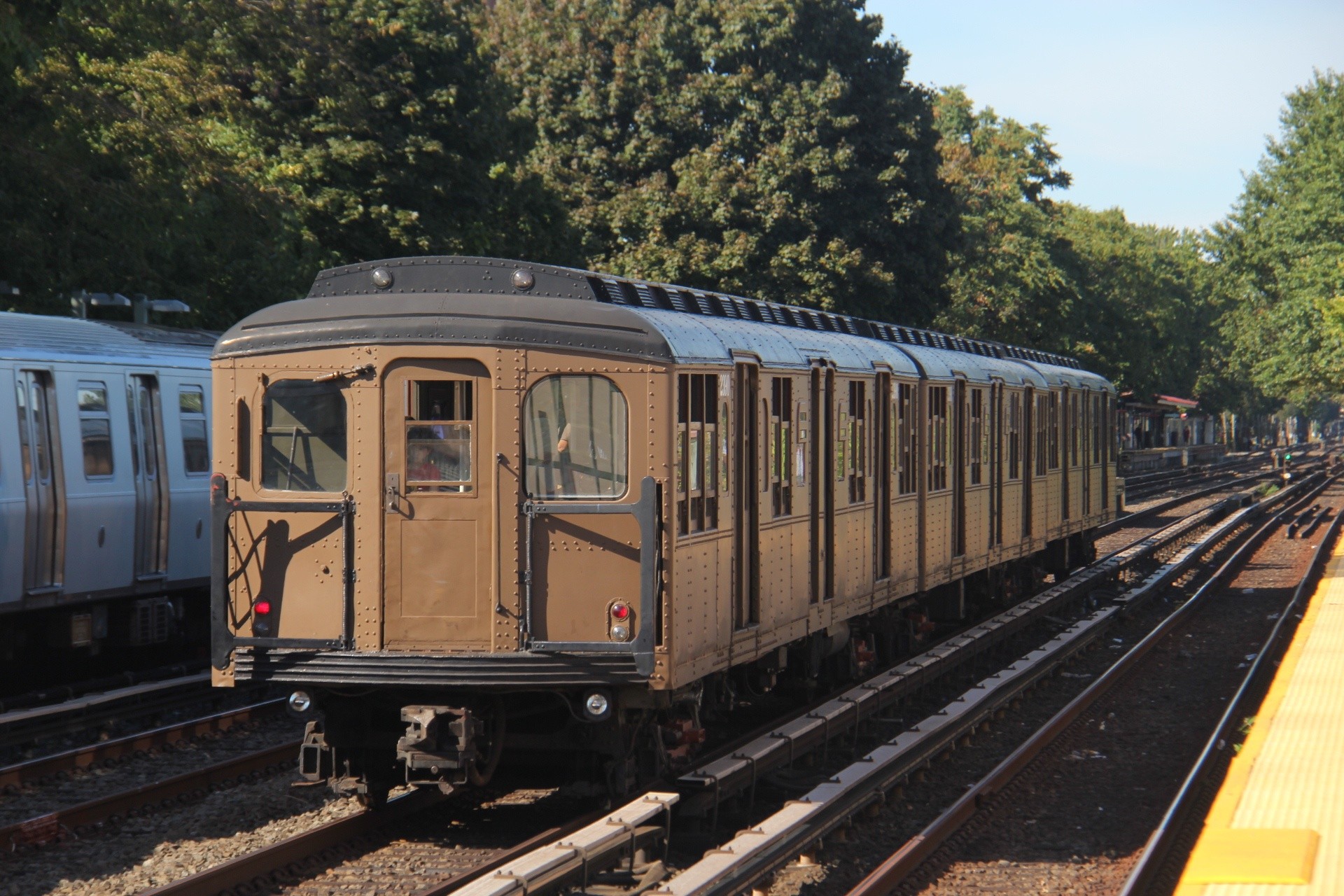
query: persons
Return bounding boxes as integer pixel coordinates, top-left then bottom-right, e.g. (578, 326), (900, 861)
(550, 404), (579, 453)
(1134, 423), (1144, 449)
(402, 417), (458, 494)
(1183, 425), (1190, 446)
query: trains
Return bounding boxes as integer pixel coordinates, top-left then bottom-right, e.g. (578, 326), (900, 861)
(210, 257), (1128, 813)
(0, 292), (229, 673)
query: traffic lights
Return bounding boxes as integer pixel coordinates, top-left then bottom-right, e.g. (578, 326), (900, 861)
(1283, 453), (1291, 474)
